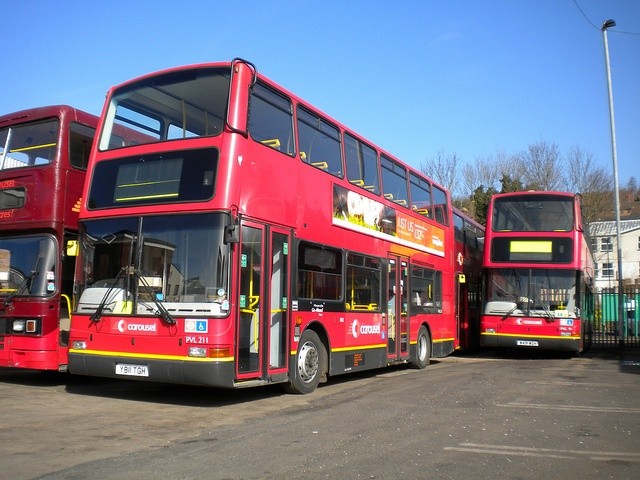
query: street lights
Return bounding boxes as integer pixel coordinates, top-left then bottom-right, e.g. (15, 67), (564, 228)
(601, 17), (626, 343)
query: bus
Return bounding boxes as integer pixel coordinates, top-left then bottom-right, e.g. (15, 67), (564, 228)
(451, 204), (485, 349)
(0, 105), (100, 374)
(481, 190), (595, 355)
(69, 58), (459, 395)
